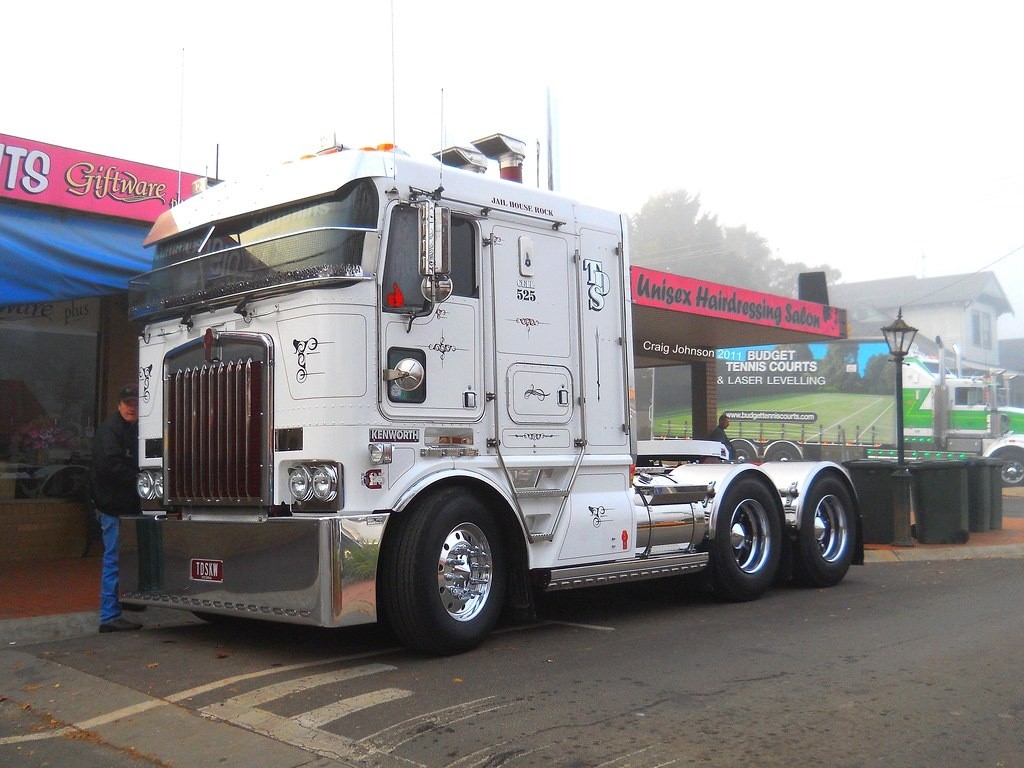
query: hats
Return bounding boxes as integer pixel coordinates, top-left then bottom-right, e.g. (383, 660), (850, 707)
(119, 385), (139, 402)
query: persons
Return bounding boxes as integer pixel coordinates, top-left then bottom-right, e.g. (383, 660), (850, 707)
(711, 416), (736, 456)
(89, 381), (148, 633)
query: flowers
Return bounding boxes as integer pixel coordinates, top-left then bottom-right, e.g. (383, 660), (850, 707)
(11, 414), (74, 450)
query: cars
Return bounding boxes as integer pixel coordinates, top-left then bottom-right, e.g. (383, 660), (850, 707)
(981, 432), (1024, 488)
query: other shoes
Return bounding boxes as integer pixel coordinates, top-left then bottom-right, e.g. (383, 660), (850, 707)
(98, 618), (144, 633)
(122, 602), (147, 612)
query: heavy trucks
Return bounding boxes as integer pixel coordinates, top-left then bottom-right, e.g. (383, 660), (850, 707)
(126, 137), (868, 658)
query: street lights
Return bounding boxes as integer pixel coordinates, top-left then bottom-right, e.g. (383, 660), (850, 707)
(880, 306), (920, 469)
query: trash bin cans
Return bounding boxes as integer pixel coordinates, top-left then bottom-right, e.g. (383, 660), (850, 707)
(906, 458), (971, 545)
(969, 457), (1007, 532)
(841, 458), (898, 544)
